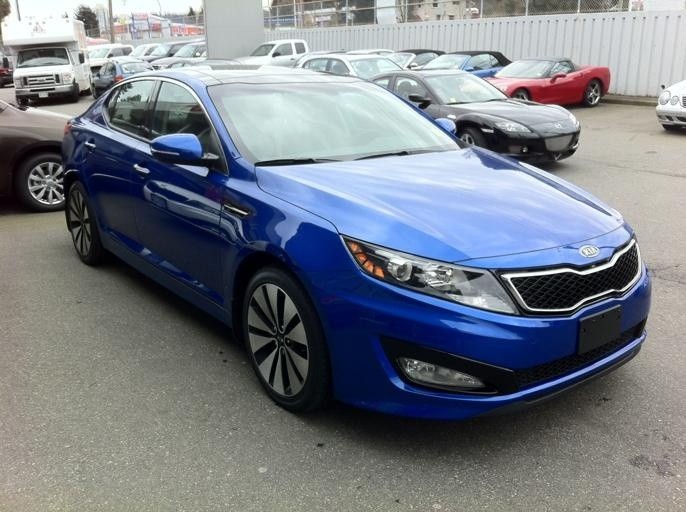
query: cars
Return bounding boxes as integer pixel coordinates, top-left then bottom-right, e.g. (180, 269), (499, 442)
(58, 67), (652, 418)
